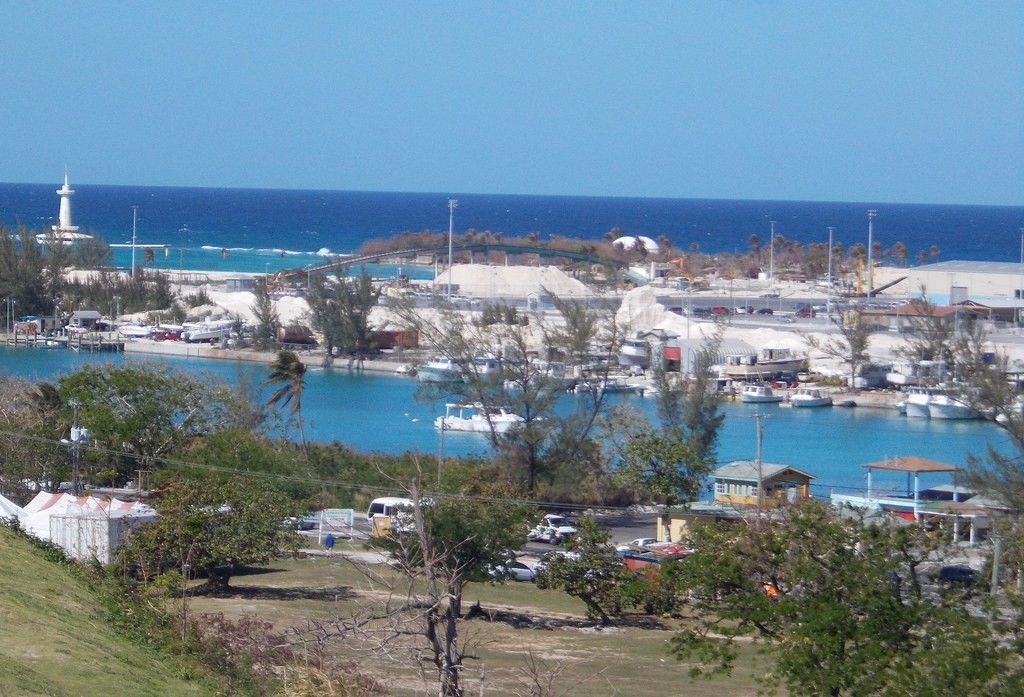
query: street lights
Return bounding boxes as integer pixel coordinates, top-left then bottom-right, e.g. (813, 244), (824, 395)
(446, 198), (460, 304)
(826, 226), (836, 296)
(867, 208), (878, 298)
(769, 220), (777, 285)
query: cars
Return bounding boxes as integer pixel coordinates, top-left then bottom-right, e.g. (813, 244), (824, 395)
(617, 537), (661, 552)
(928, 564), (985, 587)
(488, 561), (548, 583)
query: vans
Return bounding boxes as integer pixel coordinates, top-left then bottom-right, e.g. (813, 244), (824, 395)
(525, 514), (578, 544)
(367, 496), (425, 533)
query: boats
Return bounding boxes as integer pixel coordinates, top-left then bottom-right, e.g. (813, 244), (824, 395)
(431, 399), (528, 434)
(397, 349), (1024, 426)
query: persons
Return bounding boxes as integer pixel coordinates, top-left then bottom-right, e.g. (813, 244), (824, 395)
(325, 532), (335, 559)
